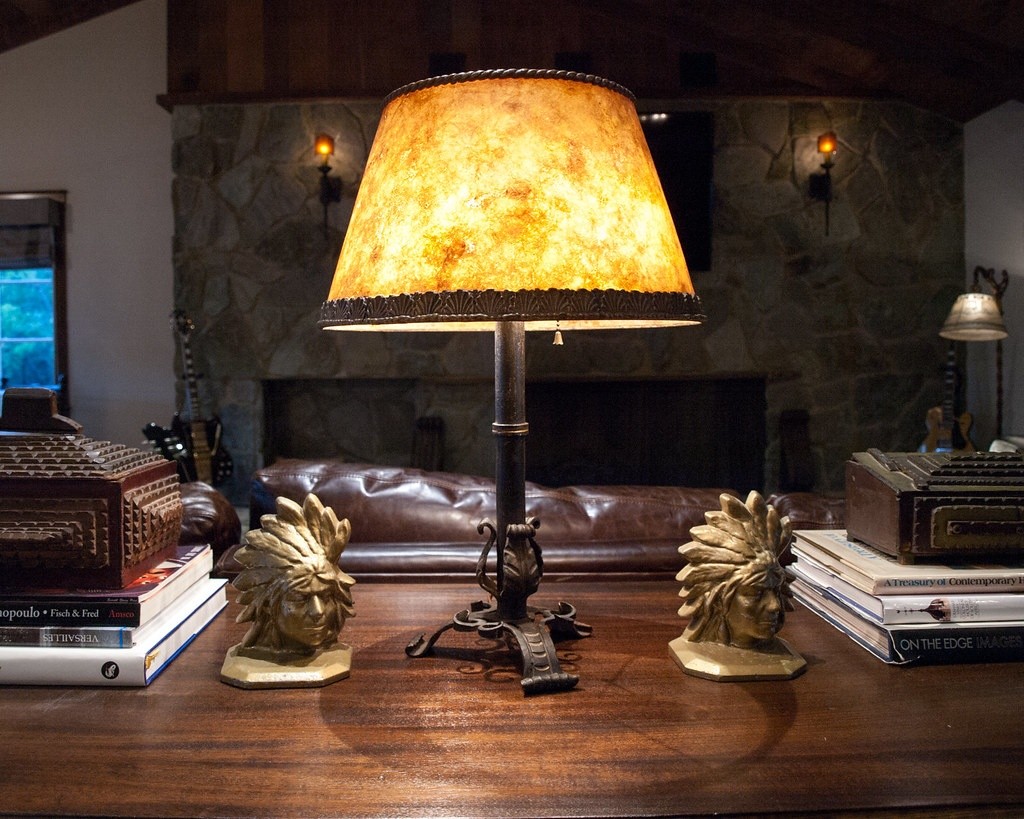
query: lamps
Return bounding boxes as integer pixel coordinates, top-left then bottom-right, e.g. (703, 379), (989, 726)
(315, 133), (341, 237)
(807, 131), (839, 223)
(317, 66), (707, 698)
(939, 265), (1011, 441)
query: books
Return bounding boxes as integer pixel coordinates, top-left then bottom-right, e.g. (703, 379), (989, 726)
(786, 529), (1024, 666)
(0, 544), (230, 685)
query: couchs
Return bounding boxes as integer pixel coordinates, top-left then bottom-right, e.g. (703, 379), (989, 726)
(214, 454), (846, 581)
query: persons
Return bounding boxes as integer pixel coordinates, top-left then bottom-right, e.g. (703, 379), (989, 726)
(668, 490), (808, 679)
(221, 494), (357, 688)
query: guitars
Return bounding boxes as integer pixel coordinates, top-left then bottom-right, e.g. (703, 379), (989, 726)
(167, 308), (234, 486)
(143, 422), (184, 472)
(915, 339), (976, 454)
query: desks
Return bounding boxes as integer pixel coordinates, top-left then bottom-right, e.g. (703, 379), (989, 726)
(0, 583), (1024, 818)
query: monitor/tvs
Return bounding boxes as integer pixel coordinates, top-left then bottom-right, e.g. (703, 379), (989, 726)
(636, 109), (714, 272)
